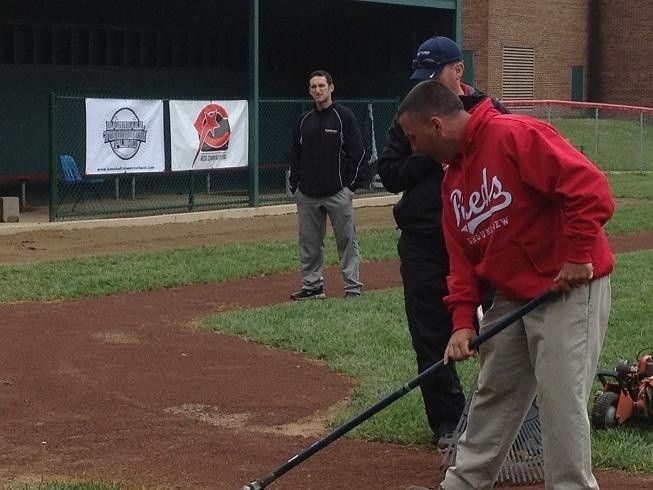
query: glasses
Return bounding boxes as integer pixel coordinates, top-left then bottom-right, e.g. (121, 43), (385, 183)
(412, 56), (460, 68)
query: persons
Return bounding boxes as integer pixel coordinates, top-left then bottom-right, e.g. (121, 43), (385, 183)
(287, 71), (366, 298)
(397, 79), (617, 489)
(378, 36), (510, 454)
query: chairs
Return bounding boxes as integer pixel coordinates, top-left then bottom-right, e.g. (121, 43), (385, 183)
(59, 155), (105, 212)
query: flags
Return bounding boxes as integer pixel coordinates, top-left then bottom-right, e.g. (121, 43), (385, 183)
(84, 97), (164, 176)
(168, 98), (248, 172)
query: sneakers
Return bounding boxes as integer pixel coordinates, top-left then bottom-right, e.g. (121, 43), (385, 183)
(437, 437), (454, 452)
(345, 292), (360, 299)
(291, 286), (325, 300)
(410, 486), (442, 490)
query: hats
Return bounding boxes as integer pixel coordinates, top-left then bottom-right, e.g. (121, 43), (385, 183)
(410, 34), (463, 81)
